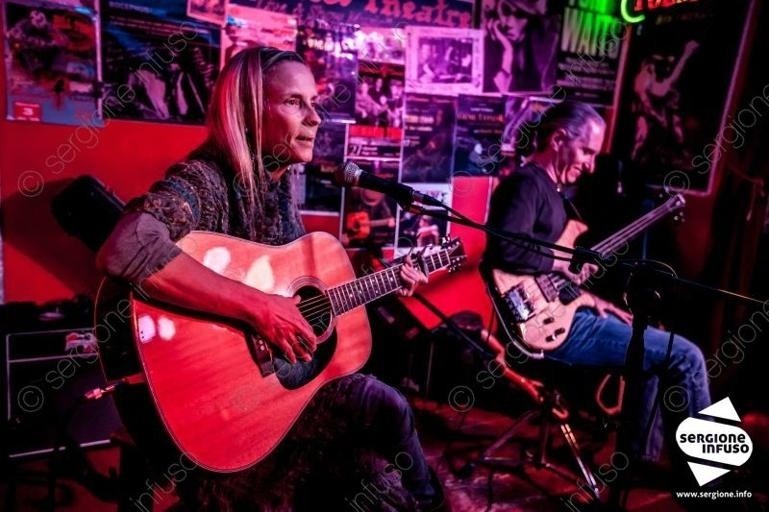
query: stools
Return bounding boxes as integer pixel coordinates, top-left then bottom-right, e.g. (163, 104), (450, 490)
(456, 342), (605, 502)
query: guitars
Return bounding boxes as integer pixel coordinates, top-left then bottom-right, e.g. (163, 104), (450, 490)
(480, 193), (685, 354)
(95, 228), (465, 477)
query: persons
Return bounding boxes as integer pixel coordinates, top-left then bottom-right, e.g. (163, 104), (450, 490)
(98, 46), (452, 511)
(484, 0), (550, 93)
(354, 75), (404, 129)
(417, 214), (440, 246)
(415, 38), (474, 83)
(479, 100), (724, 496)
(126, 46), (216, 126)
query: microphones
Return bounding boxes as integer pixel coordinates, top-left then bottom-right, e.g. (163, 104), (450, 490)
(334, 161), (442, 207)
